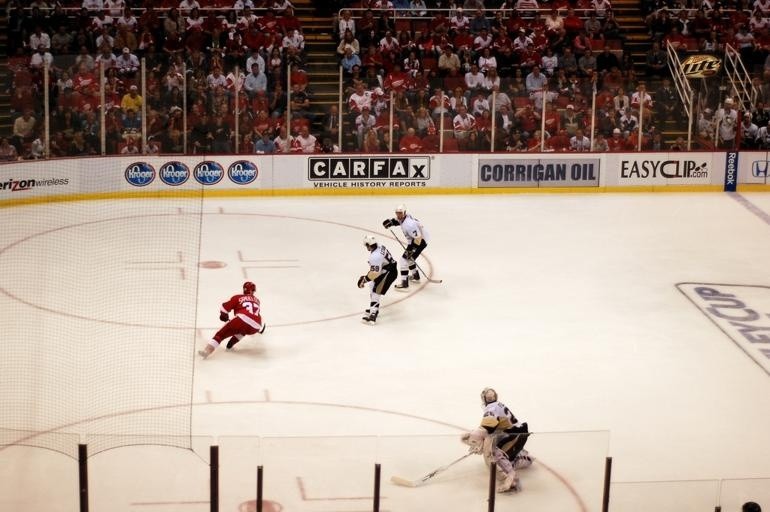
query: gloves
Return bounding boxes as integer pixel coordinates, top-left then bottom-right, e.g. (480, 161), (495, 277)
(357, 276), (366, 288)
(220, 316), (228, 321)
(260, 325), (265, 333)
(383, 219), (392, 228)
(403, 252), (411, 259)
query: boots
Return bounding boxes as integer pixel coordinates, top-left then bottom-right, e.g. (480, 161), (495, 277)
(411, 271), (420, 280)
(362, 315), (376, 322)
(394, 277), (408, 289)
(366, 309), (379, 314)
(198, 344), (215, 360)
(226, 345), (232, 350)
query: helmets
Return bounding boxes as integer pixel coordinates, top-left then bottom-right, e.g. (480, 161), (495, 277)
(243, 282), (256, 294)
(481, 388), (497, 403)
(395, 204), (409, 217)
(364, 233), (377, 246)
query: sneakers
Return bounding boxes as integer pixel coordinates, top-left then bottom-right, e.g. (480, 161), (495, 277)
(513, 456), (531, 469)
(497, 479), (521, 494)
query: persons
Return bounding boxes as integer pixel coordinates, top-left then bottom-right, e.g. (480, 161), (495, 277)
(1, 1), (769, 162)
(461, 387), (531, 493)
(382, 201), (427, 291)
(357, 236), (398, 325)
(198, 282), (261, 359)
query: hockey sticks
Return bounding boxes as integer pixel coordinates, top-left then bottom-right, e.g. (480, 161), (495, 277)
(226, 317), (266, 335)
(388, 229), (443, 284)
(390, 451), (475, 486)
